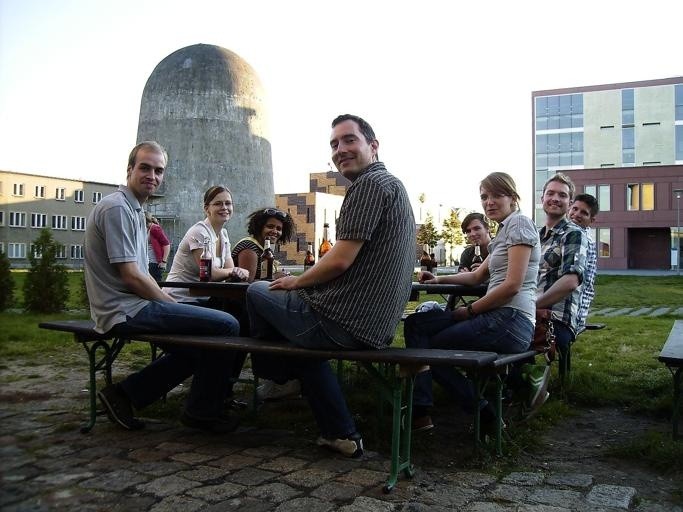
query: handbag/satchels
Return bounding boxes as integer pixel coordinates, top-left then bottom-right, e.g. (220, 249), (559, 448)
(535, 308), (556, 366)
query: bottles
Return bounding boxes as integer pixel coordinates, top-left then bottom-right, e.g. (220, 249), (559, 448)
(259, 235), (274, 281)
(199, 236), (213, 282)
(430, 244), (437, 276)
(304, 241), (315, 272)
(420, 241), (432, 283)
(317, 223), (334, 262)
(469, 243), (484, 274)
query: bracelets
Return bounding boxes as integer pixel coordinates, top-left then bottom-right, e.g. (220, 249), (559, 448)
(162, 260), (167, 263)
(466, 304), (476, 317)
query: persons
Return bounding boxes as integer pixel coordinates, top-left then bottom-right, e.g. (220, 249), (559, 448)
(246, 114), (417, 456)
(430, 252), (435, 262)
(145, 207), (172, 282)
(231, 207), (297, 284)
(419, 249), (430, 260)
(554, 192), (600, 356)
(500, 172), (588, 425)
(456, 212), (497, 274)
(160, 185), (250, 411)
(400, 171), (543, 435)
(82, 139), (240, 432)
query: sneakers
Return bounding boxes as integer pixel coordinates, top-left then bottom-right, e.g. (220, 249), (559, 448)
(180, 409), (238, 432)
(99, 385), (145, 429)
(318, 431), (363, 458)
(411, 416), (434, 432)
(257, 381), (300, 399)
(512, 391), (550, 423)
(521, 363), (551, 408)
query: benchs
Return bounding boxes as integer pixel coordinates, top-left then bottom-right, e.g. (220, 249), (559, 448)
(36, 319), (498, 493)
(655, 319), (683, 440)
(401, 311), (605, 384)
(461, 326), (585, 456)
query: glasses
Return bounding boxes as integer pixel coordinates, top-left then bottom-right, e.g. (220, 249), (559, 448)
(264, 208), (286, 217)
(208, 201), (233, 206)
